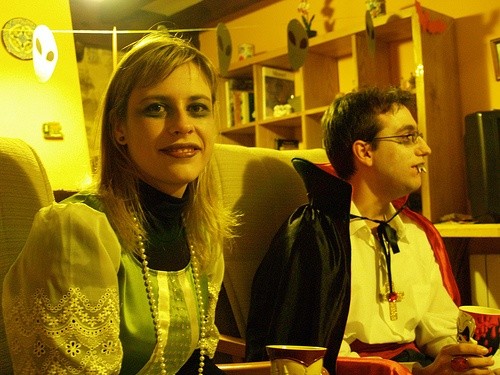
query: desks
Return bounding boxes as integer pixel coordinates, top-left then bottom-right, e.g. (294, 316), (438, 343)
(433, 218), (500, 238)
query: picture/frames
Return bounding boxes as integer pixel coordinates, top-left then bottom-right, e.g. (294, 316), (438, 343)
(491, 37), (500, 81)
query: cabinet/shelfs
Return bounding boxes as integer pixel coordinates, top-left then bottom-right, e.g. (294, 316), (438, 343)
(211, 9), (471, 306)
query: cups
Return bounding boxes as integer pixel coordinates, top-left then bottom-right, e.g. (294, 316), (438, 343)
(456, 306), (500, 368)
(238, 45), (254, 62)
(368, 0), (386, 19)
(266, 344), (326, 375)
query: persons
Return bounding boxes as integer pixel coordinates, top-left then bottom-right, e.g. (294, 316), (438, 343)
(1, 34), (246, 374)
(277, 83), (500, 375)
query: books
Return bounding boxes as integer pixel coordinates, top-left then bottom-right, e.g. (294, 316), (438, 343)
(223, 79), (255, 129)
(262, 66), (296, 120)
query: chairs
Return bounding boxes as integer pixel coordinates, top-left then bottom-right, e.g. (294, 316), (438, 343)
(205, 141), (414, 374)
(0, 136), (272, 375)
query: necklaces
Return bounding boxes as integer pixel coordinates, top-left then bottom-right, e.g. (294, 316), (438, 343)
(378, 212), (405, 321)
(132, 210), (206, 373)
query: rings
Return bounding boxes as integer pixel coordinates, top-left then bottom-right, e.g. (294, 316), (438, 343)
(449, 355), (471, 372)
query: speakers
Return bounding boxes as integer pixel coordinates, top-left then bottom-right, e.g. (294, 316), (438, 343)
(464, 109), (500, 224)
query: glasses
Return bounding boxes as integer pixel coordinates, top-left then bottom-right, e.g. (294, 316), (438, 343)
(361, 132), (424, 143)
